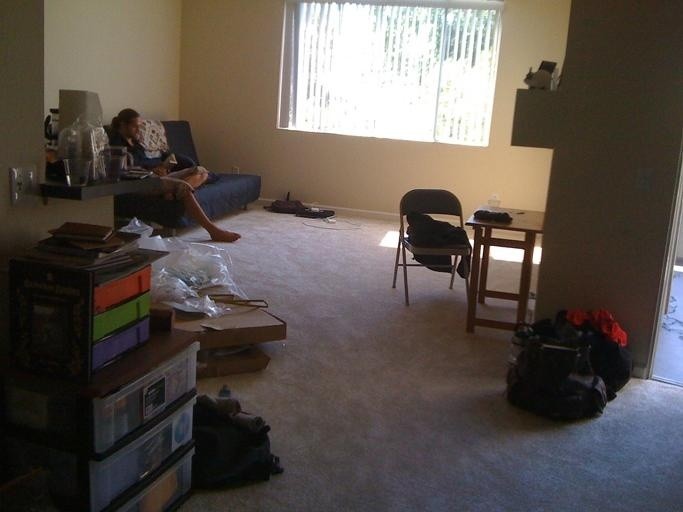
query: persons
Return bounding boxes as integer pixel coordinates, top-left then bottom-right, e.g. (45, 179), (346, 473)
(107, 107), (242, 243)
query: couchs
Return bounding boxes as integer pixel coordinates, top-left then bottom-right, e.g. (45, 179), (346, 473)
(102, 120), (262, 237)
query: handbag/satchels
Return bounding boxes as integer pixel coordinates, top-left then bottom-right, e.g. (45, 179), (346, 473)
(506, 319), (633, 421)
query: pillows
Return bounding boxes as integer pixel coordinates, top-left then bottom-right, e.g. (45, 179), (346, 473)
(167, 154), (219, 189)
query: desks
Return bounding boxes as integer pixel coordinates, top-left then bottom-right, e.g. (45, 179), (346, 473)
(465, 205), (544, 334)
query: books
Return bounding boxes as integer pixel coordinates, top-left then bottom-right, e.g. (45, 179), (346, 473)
(161, 152), (178, 171)
(122, 167), (154, 180)
(4, 220), (170, 289)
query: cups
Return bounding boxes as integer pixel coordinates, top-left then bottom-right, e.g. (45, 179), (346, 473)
(63, 157), (92, 187)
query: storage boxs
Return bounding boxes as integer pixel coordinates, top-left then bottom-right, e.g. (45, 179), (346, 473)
(1, 334), (201, 512)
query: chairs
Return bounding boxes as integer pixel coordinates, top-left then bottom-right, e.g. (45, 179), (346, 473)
(392, 189), (469, 306)
